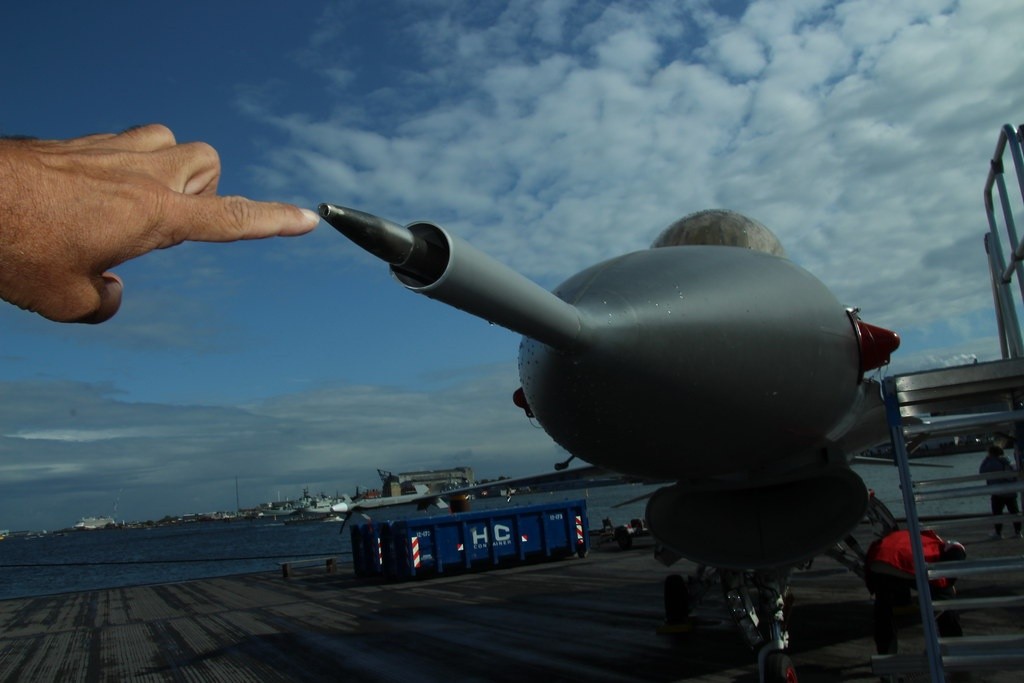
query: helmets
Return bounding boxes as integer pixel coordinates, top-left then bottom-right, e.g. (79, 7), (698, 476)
(944, 539), (966, 561)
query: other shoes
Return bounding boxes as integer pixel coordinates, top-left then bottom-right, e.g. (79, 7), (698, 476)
(1016, 533), (1023, 538)
(988, 533), (1004, 540)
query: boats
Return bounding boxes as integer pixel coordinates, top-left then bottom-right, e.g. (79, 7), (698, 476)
(284, 509), (345, 524)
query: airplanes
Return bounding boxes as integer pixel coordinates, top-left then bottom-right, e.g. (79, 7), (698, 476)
(317, 202), (1023, 683)
(252, 481), (450, 522)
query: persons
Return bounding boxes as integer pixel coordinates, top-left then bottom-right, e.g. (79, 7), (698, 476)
(864, 530), (968, 683)
(0, 122), (321, 325)
(980, 445), (1023, 538)
